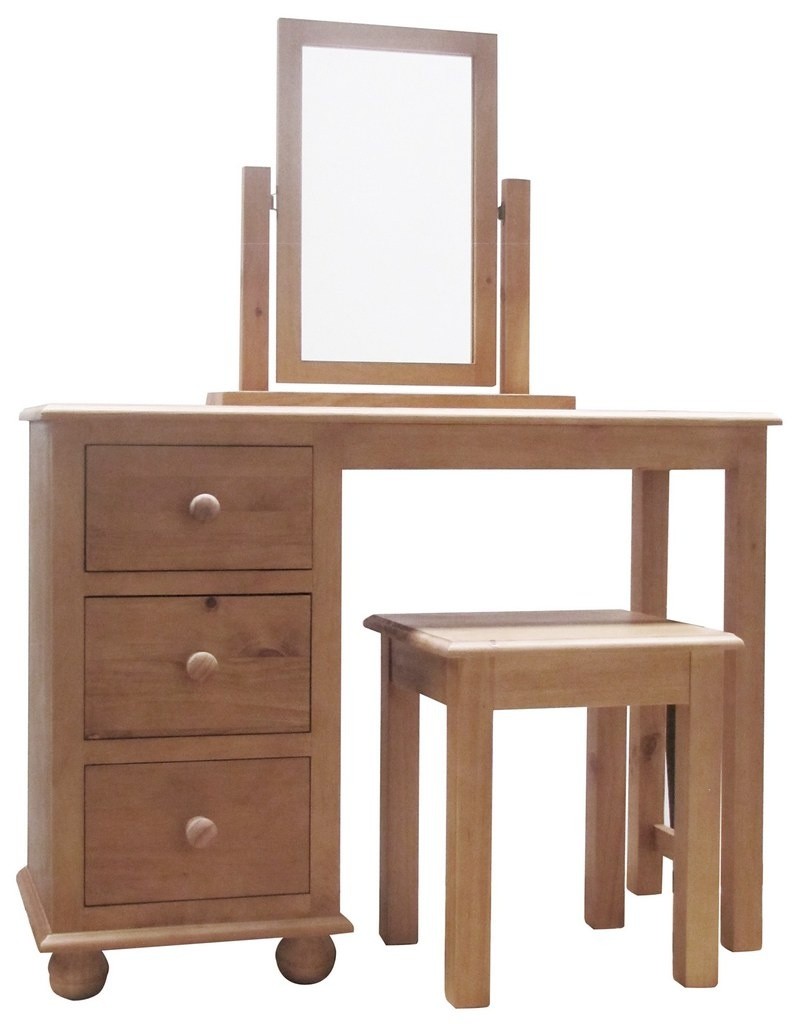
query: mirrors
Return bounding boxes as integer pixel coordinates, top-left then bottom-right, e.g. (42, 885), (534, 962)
(205, 15), (577, 406)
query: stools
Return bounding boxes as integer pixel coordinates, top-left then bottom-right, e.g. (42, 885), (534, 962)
(365, 612), (746, 1008)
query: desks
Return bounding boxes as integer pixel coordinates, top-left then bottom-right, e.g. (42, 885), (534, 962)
(18, 404), (787, 1001)
